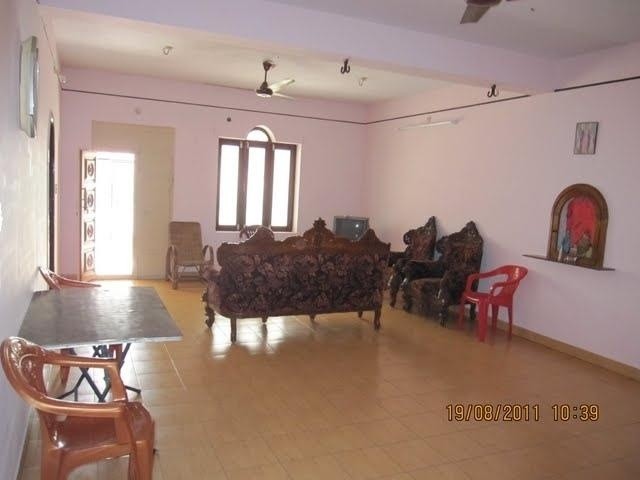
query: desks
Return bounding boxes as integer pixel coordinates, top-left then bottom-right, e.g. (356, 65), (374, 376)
(18, 286), (182, 405)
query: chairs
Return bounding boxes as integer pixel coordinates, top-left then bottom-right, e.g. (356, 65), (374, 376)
(38, 264), (123, 385)
(165, 220), (214, 290)
(1, 335), (155, 480)
(389, 217), (528, 344)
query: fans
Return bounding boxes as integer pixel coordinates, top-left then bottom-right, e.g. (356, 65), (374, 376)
(203, 60), (298, 104)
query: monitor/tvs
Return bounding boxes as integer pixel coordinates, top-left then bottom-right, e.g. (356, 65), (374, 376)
(333, 216), (368, 240)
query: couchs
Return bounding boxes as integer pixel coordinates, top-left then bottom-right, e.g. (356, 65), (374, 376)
(200, 217), (392, 345)
